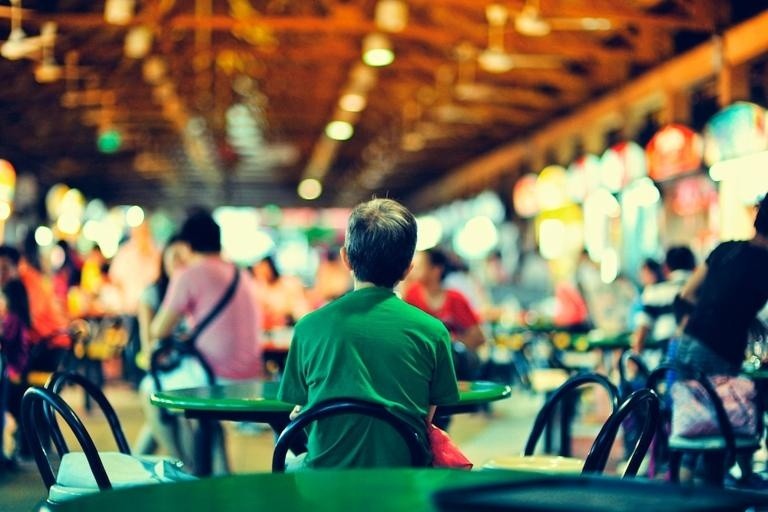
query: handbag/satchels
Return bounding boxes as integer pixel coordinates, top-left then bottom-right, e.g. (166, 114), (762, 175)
(156, 334), (181, 367)
(670, 377), (757, 437)
(429, 423), (473, 469)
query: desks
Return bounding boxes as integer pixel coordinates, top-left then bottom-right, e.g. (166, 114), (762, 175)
(50, 466), (768, 512)
(151, 381), (511, 456)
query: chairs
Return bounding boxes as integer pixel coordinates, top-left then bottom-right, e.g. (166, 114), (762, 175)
(524, 373), (619, 473)
(271, 399), (427, 472)
(21, 387), (200, 512)
(43, 372), (184, 468)
(647, 363), (762, 487)
(579, 389), (659, 477)
(618, 349), (653, 395)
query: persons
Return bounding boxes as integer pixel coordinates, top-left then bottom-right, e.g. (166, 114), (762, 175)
(400, 191), (768, 484)
(277, 193), (461, 471)
(0, 196), (352, 486)
